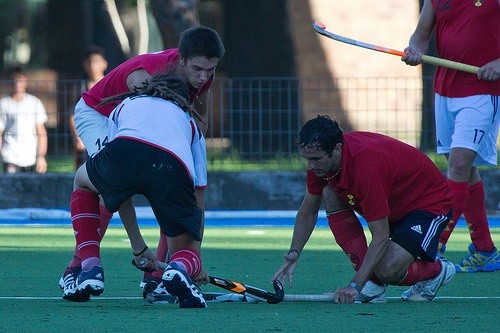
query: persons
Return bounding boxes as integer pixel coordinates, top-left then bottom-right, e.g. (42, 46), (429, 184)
(0, 69), (50, 175)
(68, 46), (109, 173)
(271, 115), (455, 304)
(62, 70), (211, 308)
(401, 0), (500, 273)
(57, 24), (226, 293)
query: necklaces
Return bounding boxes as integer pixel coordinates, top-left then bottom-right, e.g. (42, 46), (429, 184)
(321, 166), (342, 180)
(188, 88), (203, 105)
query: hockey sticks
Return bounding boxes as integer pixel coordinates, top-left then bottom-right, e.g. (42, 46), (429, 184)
(311, 18), (480, 74)
(144, 292), (337, 302)
(139, 256), (284, 304)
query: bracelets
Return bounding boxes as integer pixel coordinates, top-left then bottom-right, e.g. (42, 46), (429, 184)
(133, 246), (148, 257)
(288, 249), (300, 258)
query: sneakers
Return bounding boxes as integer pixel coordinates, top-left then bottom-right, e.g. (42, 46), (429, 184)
(435, 242), (446, 258)
(59, 266), (80, 293)
(402, 257), (456, 302)
(63, 266), (105, 302)
(140, 275), (174, 304)
(354, 286), (387, 303)
(162, 262), (208, 309)
(454, 243), (500, 272)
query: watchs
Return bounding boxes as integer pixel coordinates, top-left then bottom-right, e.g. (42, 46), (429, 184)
(349, 281), (362, 293)
